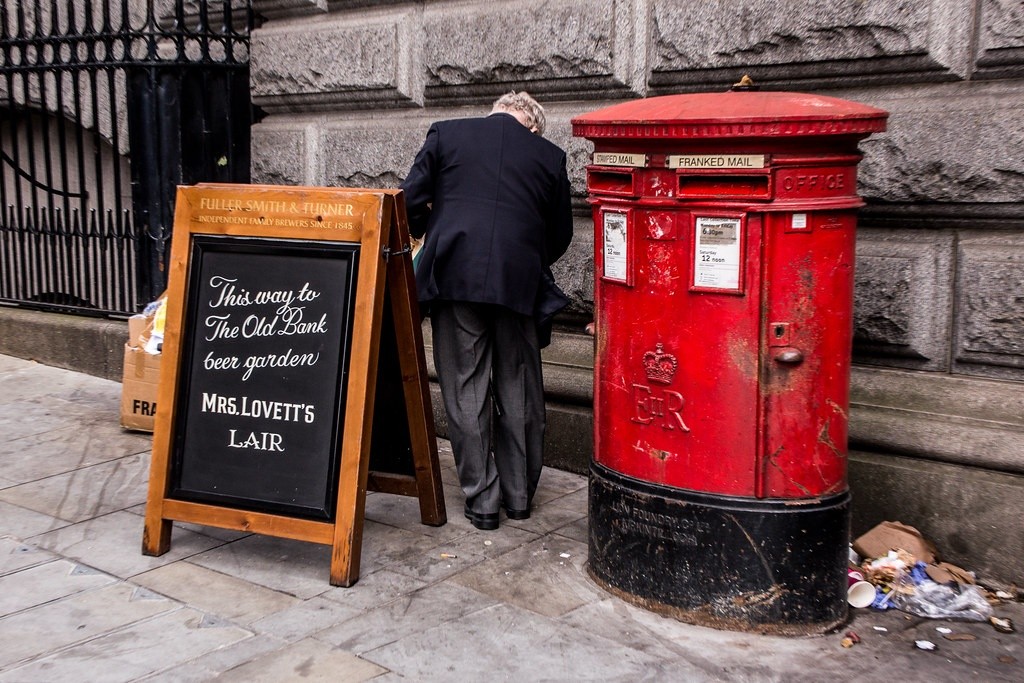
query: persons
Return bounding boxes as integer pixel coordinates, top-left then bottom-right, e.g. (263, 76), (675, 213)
(399, 91), (573, 531)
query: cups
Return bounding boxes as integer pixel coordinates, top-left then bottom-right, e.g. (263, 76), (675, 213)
(848, 568), (876, 608)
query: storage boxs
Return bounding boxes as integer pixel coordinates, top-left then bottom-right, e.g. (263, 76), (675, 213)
(119, 313), (162, 433)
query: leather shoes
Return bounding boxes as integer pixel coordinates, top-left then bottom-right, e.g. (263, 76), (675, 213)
(500, 499), (531, 519)
(463, 504), (500, 530)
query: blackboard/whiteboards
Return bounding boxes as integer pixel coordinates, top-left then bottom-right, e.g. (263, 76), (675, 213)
(148, 185), (435, 545)
